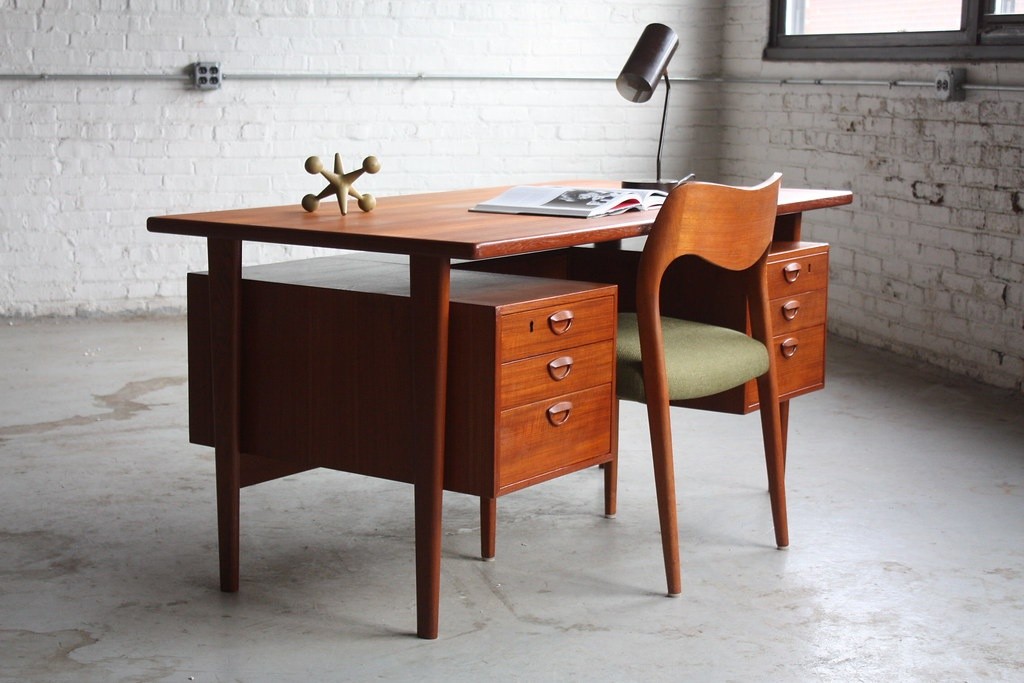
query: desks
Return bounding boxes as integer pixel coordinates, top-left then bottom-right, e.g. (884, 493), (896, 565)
(146, 180), (855, 639)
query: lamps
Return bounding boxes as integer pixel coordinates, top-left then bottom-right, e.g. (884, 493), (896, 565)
(615, 21), (698, 193)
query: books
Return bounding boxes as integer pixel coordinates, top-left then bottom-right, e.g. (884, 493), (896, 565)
(467, 185), (669, 219)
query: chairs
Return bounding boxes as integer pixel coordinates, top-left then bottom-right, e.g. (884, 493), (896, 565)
(480, 170), (789, 596)
